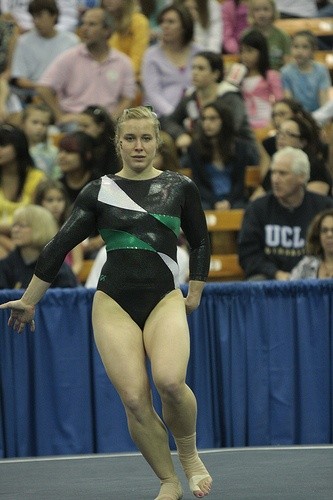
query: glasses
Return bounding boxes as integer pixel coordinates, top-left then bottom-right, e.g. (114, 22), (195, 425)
(276, 126), (301, 139)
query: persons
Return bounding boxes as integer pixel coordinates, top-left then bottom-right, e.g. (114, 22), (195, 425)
(0, 0), (333, 288)
(0, 106), (213, 500)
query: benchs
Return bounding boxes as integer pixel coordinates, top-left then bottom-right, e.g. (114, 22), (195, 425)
(53, 14), (333, 280)
